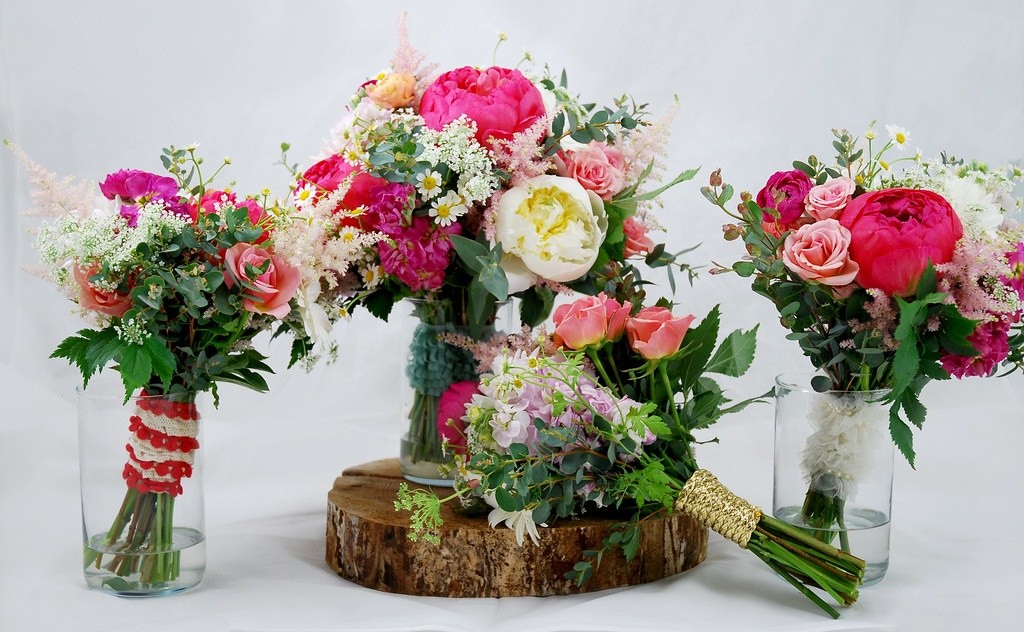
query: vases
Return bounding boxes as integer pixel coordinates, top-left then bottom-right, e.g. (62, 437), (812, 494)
(770, 367), (894, 586)
(395, 292), (517, 485)
(72, 383), (207, 598)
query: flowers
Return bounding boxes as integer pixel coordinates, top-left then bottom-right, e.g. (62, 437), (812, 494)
(698, 121), (1024, 558)
(389, 288), (867, 622)
(272, 6), (708, 461)
(7, 134), (398, 586)
